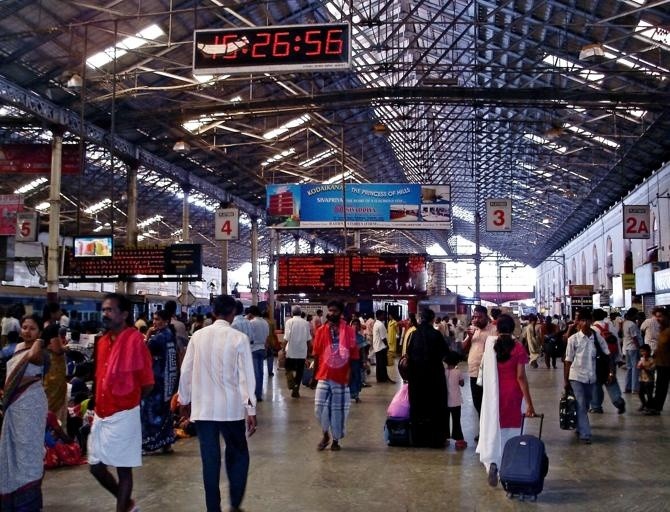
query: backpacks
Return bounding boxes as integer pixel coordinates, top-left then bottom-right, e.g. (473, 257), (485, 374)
(593, 323), (619, 357)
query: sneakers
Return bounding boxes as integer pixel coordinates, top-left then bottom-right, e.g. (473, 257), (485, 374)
(455, 440), (467, 448)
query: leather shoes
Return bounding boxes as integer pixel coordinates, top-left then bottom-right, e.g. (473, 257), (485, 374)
(257, 397), (262, 402)
(580, 438), (592, 444)
(638, 405), (661, 415)
(589, 407), (604, 413)
(617, 399), (625, 415)
(362, 382), (368, 387)
(575, 428), (580, 435)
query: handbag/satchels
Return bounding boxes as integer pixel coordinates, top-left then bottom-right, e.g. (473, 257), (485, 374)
(399, 354), (409, 380)
(594, 354), (610, 379)
(560, 391), (579, 430)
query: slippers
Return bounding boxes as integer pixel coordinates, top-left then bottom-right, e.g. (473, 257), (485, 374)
(331, 443), (341, 451)
(318, 437), (329, 451)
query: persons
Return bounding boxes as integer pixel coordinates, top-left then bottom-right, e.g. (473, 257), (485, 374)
(81, 292), (157, 512)
(0, 312), (51, 509)
(562, 307), (670, 415)
(475, 314), (538, 490)
(396, 312), (465, 354)
(405, 308), (456, 449)
(0, 300), (94, 452)
(443, 351), (467, 448)
(463, 306), (497, 443)
(174, 294), (260, 512)
(281, 299), (399, 404)
(231, 300), (276, 403)
(312, 300), (360, 452)
(135, 300), (216, 451)
(564, 308), (613, 444)
(489, 308), (574, 369)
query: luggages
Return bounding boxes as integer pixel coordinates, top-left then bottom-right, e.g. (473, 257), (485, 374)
(499, 412), (550, 502)
(384, 380), (412, 447)
(303, 367), (315, 387)
